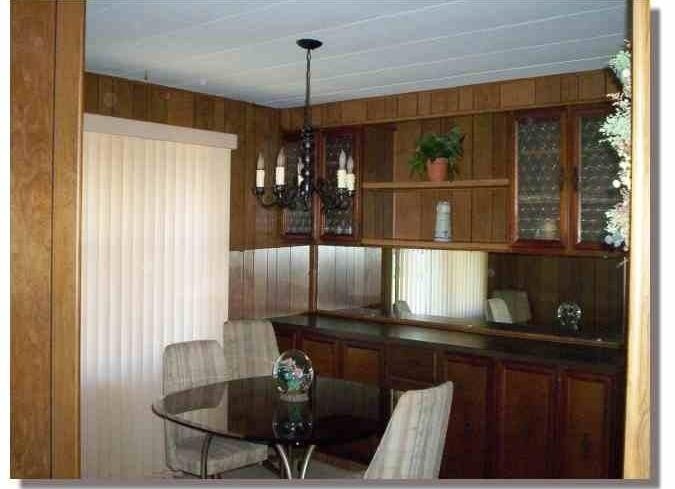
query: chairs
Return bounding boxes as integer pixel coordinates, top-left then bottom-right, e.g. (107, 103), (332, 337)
(361, 380), (454, 479)
(161, 339), (270, 481)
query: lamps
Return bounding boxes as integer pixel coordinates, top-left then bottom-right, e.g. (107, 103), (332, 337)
(248, 37), (355, 221)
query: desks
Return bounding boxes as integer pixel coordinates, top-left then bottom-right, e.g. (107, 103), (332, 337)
(153, 374), (399, 479)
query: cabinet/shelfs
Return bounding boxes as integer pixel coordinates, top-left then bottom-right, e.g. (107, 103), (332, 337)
(269, 307), (633, 479)
(276, 68), (631, 250)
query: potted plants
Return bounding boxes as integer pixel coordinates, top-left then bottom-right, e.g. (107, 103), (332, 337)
(406, 124), (464, 184)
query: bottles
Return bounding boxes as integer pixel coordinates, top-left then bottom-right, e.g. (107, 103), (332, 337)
(435, 198), (453, 242)
